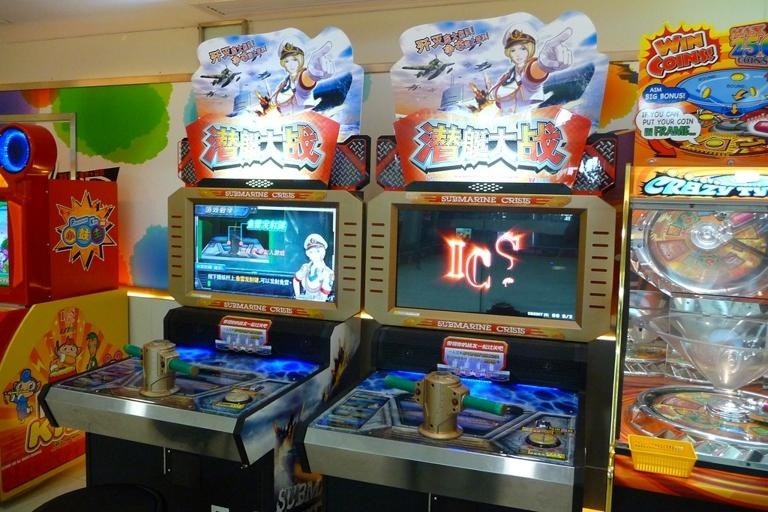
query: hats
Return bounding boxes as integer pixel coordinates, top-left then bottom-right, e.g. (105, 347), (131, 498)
(504, 24), (535, 46)
(278, 39), (307, 59)
(304, 233), (328, 251)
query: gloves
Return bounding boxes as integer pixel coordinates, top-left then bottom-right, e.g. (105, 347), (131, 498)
(539, 28), (573, 73)
(306, 41), (334, 82)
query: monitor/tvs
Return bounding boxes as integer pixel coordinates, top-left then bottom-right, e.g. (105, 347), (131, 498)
(0, 201), (9, 286)
(193, 202), (338, 303)
(630, 209), (768, 299)
(395, 206), (581, 322)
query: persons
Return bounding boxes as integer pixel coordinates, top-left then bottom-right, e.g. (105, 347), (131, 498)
(291, 231), (335, 303)
(262, 35), (336, 117)
(480, 20), (574, 115)
